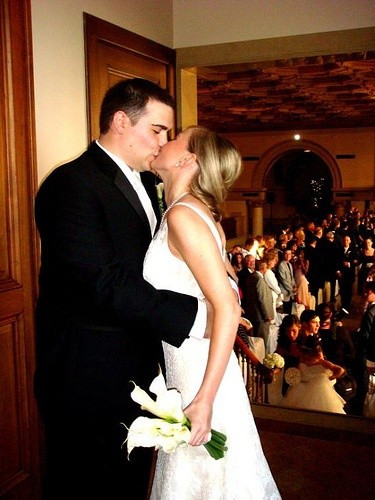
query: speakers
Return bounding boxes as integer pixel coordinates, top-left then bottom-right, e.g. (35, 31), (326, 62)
(267, 192), (275, 203)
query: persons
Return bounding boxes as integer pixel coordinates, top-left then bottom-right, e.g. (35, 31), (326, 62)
(33, 78), (240, 500)
(142, 126), (282, 500)
(228, 204), (375, 415)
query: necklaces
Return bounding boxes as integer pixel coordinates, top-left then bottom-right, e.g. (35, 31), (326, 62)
(160, 193), (189, 222)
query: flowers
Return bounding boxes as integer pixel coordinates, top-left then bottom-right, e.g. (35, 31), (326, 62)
(120, 362), (229, 462)
(285, 369), (303, 387)
(261, 352), (286, 372)
